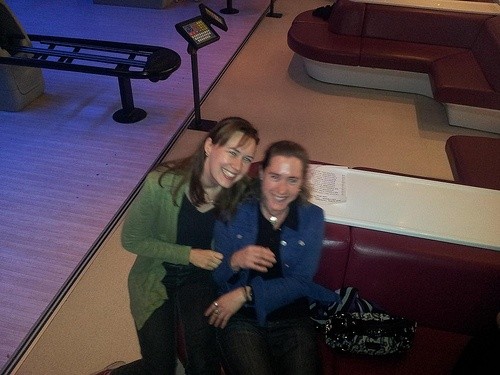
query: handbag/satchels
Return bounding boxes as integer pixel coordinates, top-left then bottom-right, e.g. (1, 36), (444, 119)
(323, 311), (418, 359)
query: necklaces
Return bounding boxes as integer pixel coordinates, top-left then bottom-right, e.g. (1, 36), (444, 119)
(264, 204), (285, 224)
(203, 192), (214, 204)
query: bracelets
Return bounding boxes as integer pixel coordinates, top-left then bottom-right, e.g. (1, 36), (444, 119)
(242, 285), (250, 304)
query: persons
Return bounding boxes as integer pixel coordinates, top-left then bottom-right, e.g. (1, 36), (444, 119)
(90, 116), (260, 375)
(206, 141), (342, 375)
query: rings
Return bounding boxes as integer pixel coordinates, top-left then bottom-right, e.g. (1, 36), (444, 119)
(212, 301), (219, 307)
(214, 309), (221, 315)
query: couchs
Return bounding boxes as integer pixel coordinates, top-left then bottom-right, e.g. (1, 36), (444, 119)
(176, 0), (500, 375)
